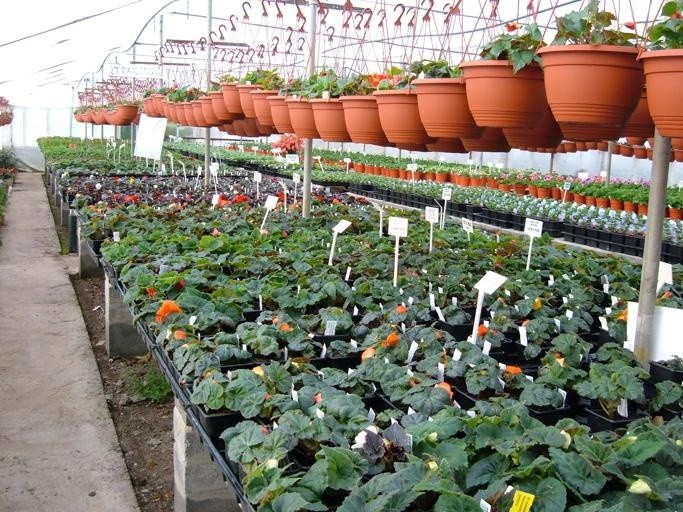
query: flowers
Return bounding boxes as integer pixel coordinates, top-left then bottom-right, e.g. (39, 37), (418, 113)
(538, 198), (550, 218)
(448, 341), (522, 397)
(361, 333), (404, 383)
(280, 399), (343, 463)
(647, 0), (682, 49)
(474, 188), (481, 206)
(354, 309), (401, 338)
(611, 210), (626, 233)
(478, 22), (546, 74)
(575, 178), (583, 194)
(586, 206), (599, 229)
(549, 2), (645, 45)
(292, 370), (375, 409)
(222, 421), (278, 463)
(413, 180), (420, 195)
(311, 190), (368, 226)
(595, 175), (608, 198)
(481, 187), (490, 208)
(523, 314), (564, 361)
(522, 195), (537, 217)
(60, 177), (311, 227)
(467, 453), (567, 512)
(451, 184), (455, 203)
(563, 201), (574, 224)
(550, 200), (563, 222)
(152, 301), (186, 342)
(657, 282), (681, 307)
(395, 178), (400, 192)
(455, 185), (460, 204)
(465, 398), (563, 453)
(278, 85), (293, 96)
(445, 184), (451, 188)
(392, 301), (433, 323)
(510, 168), (516, 184)
(476, 319), (508, 350)
(583, 178), (594, 196)
(254, 309), (306, 342)
(498, 191), (512, 213)
(556, 176), (565, 191)
(470, 187), (474, 204)
(242, 464), (314, 512)
(574, 202), (586, 226)
(460, 187), (466, 204)
(466, 187), (470, 204)
(531, 170), (541, 186)
(341, 74), (387, 96)
(426, 181), (432, 198)
(243, 366), (290, 419)
(433, 181), (437, 199)
(389, 178), (395, 191)
(437, 183), (444, 200)
(608, 177), (623, 200)
(187, 86), (200, 100)
(378, 67), (415, 90)
(669, 219), (683, 244)
(599, 209), (611, 232)
(622, 177), (636, 201)
(406, 180), (413, 195)
(401, 179), (406, 193)
(314, 409), (466, 512)
(562, 419), (682, 512)
(638, 215), (647, 238)
(490, 189), (498, 210)
(194, 368), (248, 412)
(403, 323), (453, 368)
(610, 299), (627, 343)
(383, 368), (453, 411)
(624, 212), (638, 236)
(565, 176), (574, 192)
(516, 168), (524, 184)
(7, 167), (17, 175)
(420, 180), (426, 196)
(287, 76), (310, 98)
(499, 168), (509, 183)
(543, 174), (553, 188)
(513, 194), (522, 215)
(634, 178), (651, 203)
(563, 306), (594, 332)
(524, 169), (531, 185)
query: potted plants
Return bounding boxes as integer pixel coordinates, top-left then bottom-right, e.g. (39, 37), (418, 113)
(155, 88), (165, 117)
(439, 305), (473, 341)
(380, 155), (383, 175)
(386, 156), (389, 176)
(495, 168), (499, 189)
(187, 309), (215, 341)
(420, 159), (424, 179)
(354, 152), (357, 172)
(78, 226), (572, 317)
(488, 167), (495, 189)
(284, 341), (324, 370)
(393, 157), (399, 178)
(363, 174), (368, 189)
(523, 365), (574, 426)
(650, 355), (683, 385)
(0, 111), (11, 126)
(538, 181), (546, 198)
(249, 68), (285, 125)
(415, 159), (420, 181)
(481, 165), (488, 186)
(503, 181), (510, 192)
(368, 154), (374, 175)
(407, 158), (412, 179)
(146, 88), (161, 117)
(372, 175), (378, 191)
(169, 343), (218, 383)
(38, 134), (306, 181)
(174, 89), (188, 126)
(358, 172), (362, 190)
(460, 163), (462, 185)
(199, 91), (224, 124)
(209, 84), (236, 120)
(612, 141), (620, 154)
(181, 86), (198, 126)
(427, 159), (433, 180)
(468, 165), (470, 186)
(389, 157), (393, 177)
(653, 381), (682, 414)
(664, 188), (669, 218)
(348, 152), (352, 168)
(566, 192), (570, 202)
(378, 176), (383, 194)
(306, 306), (354, 344)
(368, 175), (372, 190)
(462, 163), (468, 186)
(150, 87), (162, 114)
(529, 182), (536, 196)
(141, 308), (152, 333)
(236, 70), (265, 117)
(167, 88), (179, 122)
(411, 59), (485, 138)
(141, 89), (152, 116)
(220, 74), (243, 113)
(433, 161), (435, 181)
(552, 178), (560, 199)
(161, 91), (170, 118)
(470, 165), (481, 187)
(374, 155), (380, 175)
(451, 163), (456, 183)
(362, 153), (365, 173)
(438, 161), (447, 181)
(74, 99), (141, 125)
(661, 218), (669, 252)
(310, 149), (348, 186)
(354, 172), (358, 189)
(352, 152), (354, 168)
(325, 339), (360, 370)
(424, 160), (427, 180)
(435, 161), (438, 181)
(349, 172), (354, 188)
(447, 163), (451, 182)
(4, 175), (13, 186)
(456, 164), (460, 185)
(365, 154), (368, 174)
(216, 309), (252, 334)
(572, 247), (641, 302)
(219, 335), (253, 369)
(574, 344), (651, 432)
(253, 338), (290, 365)
(383, 155), (386, 175)
(669, 184), (682, 220)
(399, 157), (407, 179)
(357, 152), (362, 173)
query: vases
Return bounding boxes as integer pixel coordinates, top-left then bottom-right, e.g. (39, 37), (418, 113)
(413, 195), (419, 202)
(579, 329), (600, 346)
(376, 393), (383, 407)
(624, 236), (638, 246)
(599, 231), (610, 241)
(522, 216), (535, 224)
(460, 204), (466, 211)
(639, 204), (647, 215)
(289, 451), (312, 473)
(499, 184), (503, 189)
(433, 199), (437, 205)
(524, 185), (528, 188)
(255, 414), (269, 426)
(670, 138), (683, 150)
(267, 96), (294, 133)
(674, 151), (682, 161)
(382, 177), (389, 190)
(537, 218), (550, 229)
(466, 205), (470, 213)
(513, 215), (522, 223)
(487, 351), (505, 358)
(309, 99), (349, 141)
(390, 191), (395, 196)
(596, 198), (600, 206)
(153, 329), (157, 339)
(437, 200), (445, 206)
(583, 196), (585, 203)
(190, 101), (210, 126)
(576, 143), (587, 151)
(600, 197), (607, 208)
(620, 144), (633, 156)
(623, 91), (654, 136)
(531, 346), (548, 361)
(516, 184), (524, 194)
(638, 238), (644, 247)
(647, 149), (653, 160)
(536, 186), (538, 197)
(401, 193), (407, 199)
(536, 45), (644, 142)
(223, 123), (235, 134)
(610, 200), (612, 208)
(597, 142), (608, 151)
(416, 318), (435, 328)
(456, 204), (460, 211)
(633, 203), (638, 213)
(256, 118), (272, 136)
(545, 148), (554, 152)
(573, 193), (575, 201)
(459, 127), (511, 152)
(607, 200), (610, 207)
(454, 379), (476, 407)
(426, 197), (433, 204)
(562, 223), (574, 233)
(499, 212), (512, 220)
(490, 210), (499, 218)
(621, 201), (624, 210)
(575, 195), (583, 204)
(550, 222), (570, 230)
(373, 91), (437, 143)
(183, 383), (194, 399)
(668, 243), (683, 255)
(624, 201), (633, 211)
(555, 146), (559, 152)
(447, 201), (451, 209)
(241, 118), (259, 136)
(470, 205), (474, 213)
(426, 137), (462, 153)
(384, 396), (398, 413)
(382, 190), (390, 196)
(574, 225), (586, 235)
(632, 147), (646, 158)
(239, 462), (247, 485)
(511, 184), (515, 189)
(13, 176), (16, 184)
(407, 195), (413, 201)
(638, 49), (682, 137)
(565, 143), (577, 152)
(502, 109), (554, 147)
(546, 188), (552, 198)
(586, 196), (593, 205)
(560, 191), (564, 199)
(460, 60), (547, 128)
(537, 147), (544, 152)
(669, 151), (674, 161)
(451, 202), (456, 210)
(339, 96), (387, 143)
(593, 199), (596, 206)
(627, 137), (645, 144)
(527, 147), (536, 151)
(559, 144), (564, 152)
(586, 228), (598, 238)
(647, 138), (654, 148)
(520, 146), (527, 150)
(395, 192), (400, 197)
(571, 193), (573, 201)
(474, 206), (482, 213)
(612, 199), (621, 210)
(219, 125), (225, 131)
(396, 144), (424, 151)
(310, 70), (341, 98)
(196, 407), (239, 446)
(419, 197), (426, 202)
(233, 120), (246, 136)
(611, 233), (624, 244)
(482, 209), (490, 216)
(585, 142), (597, 148)
(223, 443), (238, 475)
(285, 98), (319, 139)
